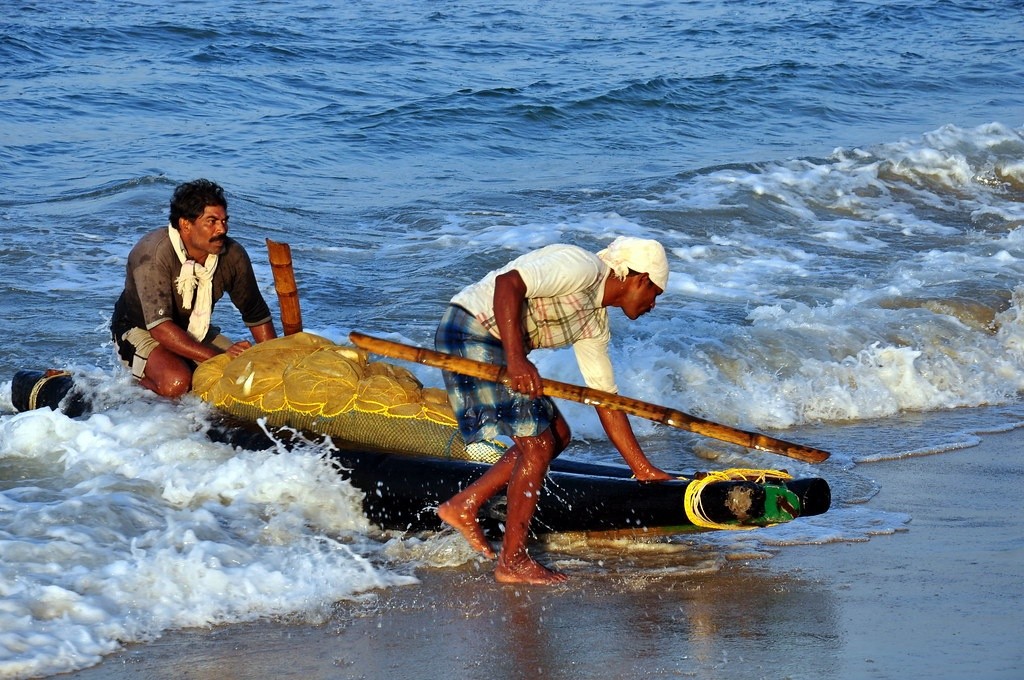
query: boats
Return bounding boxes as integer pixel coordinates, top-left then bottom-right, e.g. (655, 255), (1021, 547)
(9, 330), (833, 545)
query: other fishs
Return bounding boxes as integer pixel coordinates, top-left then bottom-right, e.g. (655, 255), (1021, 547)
(189, 331), (509, 465)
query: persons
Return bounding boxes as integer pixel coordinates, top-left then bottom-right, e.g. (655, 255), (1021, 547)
(435, 235), (669, 585)
(110, 180), (276, 401)
